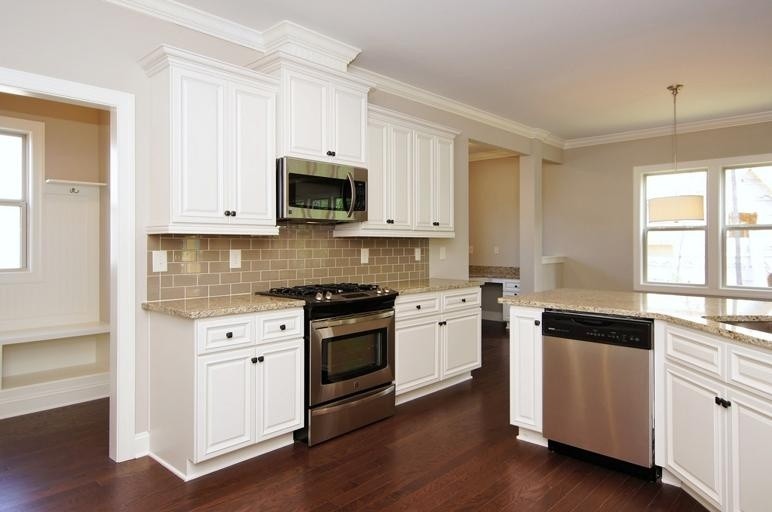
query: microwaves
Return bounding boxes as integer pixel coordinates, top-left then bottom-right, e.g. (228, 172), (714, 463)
(276, 156), (369, 225)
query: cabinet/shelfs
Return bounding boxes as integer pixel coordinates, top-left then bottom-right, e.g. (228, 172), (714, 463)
(660, 323), (772, 512)
(392, 286), (483, 406)
(147, 309), (307, 483)
(510, 306), (549, 448)
(136, 43), (280, 236)
(242, 49), (374, 168)
(332, 103), (461, 239)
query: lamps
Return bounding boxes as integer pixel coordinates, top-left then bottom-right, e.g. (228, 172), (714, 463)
(648, 83), (704, 224)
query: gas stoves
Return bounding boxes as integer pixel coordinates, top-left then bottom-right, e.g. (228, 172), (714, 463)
(254, 283), (399, 304)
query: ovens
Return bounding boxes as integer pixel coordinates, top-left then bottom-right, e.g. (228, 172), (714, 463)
(306, 308), (396, 448)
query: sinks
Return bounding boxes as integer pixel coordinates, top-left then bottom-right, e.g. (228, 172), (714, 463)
(700, 314), (772, 334)
